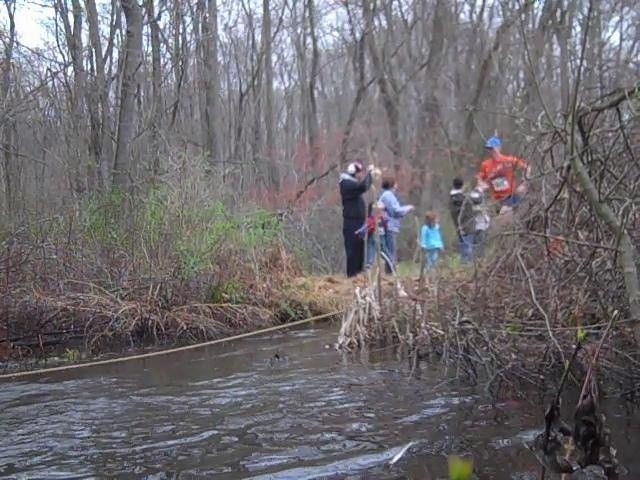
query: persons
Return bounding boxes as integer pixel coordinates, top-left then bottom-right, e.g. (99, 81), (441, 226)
(340, 161), (374, 279)
(449, 177), (472, 266)
(474, 136), (532, 216)
(354, 199), (393, 276)
(379, 175), (413, 277)
(470, 188), (490, 248)
(418, 210), (445, 272)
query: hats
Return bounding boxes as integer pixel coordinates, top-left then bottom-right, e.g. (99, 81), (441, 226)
(347, 162), (362, 174)
(484, 136), (503, 147)
